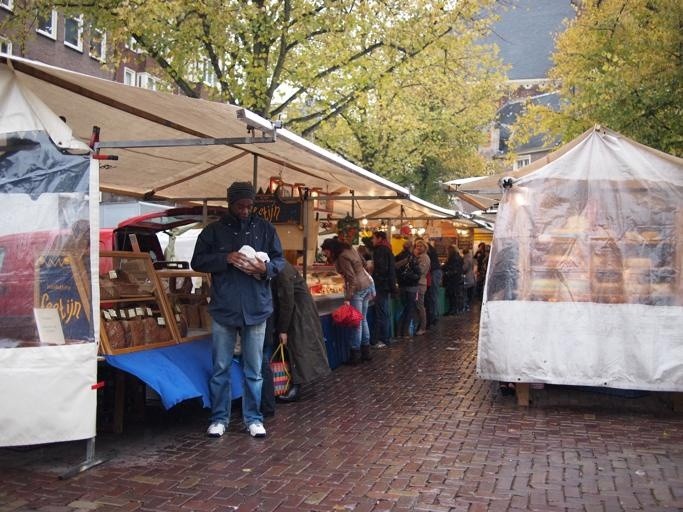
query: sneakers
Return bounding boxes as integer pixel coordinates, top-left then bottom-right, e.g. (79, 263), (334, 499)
(207, 422), (225, 437)
(247, 423), (266, 437)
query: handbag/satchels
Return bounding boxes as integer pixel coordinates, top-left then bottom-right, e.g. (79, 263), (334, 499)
(270, 343), (291, 396)
(363, 267), (376, 297)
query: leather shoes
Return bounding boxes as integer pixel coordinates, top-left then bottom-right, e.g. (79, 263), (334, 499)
(416, 329), (425, 335)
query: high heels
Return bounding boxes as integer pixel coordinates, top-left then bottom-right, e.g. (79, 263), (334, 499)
(279, 385), (299, 401)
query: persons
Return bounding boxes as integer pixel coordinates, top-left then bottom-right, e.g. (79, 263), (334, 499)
(267, 254), (334, 404)
(189, 179), (285, 438)
(321, 230), (492, 367)
(71, 218), (92, 279)
(260, 324), (277, 418)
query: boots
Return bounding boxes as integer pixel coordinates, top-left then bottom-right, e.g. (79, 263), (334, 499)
(344, 345), (373, 366)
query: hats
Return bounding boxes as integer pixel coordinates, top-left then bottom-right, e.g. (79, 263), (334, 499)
(227, 182), (256, 203)
